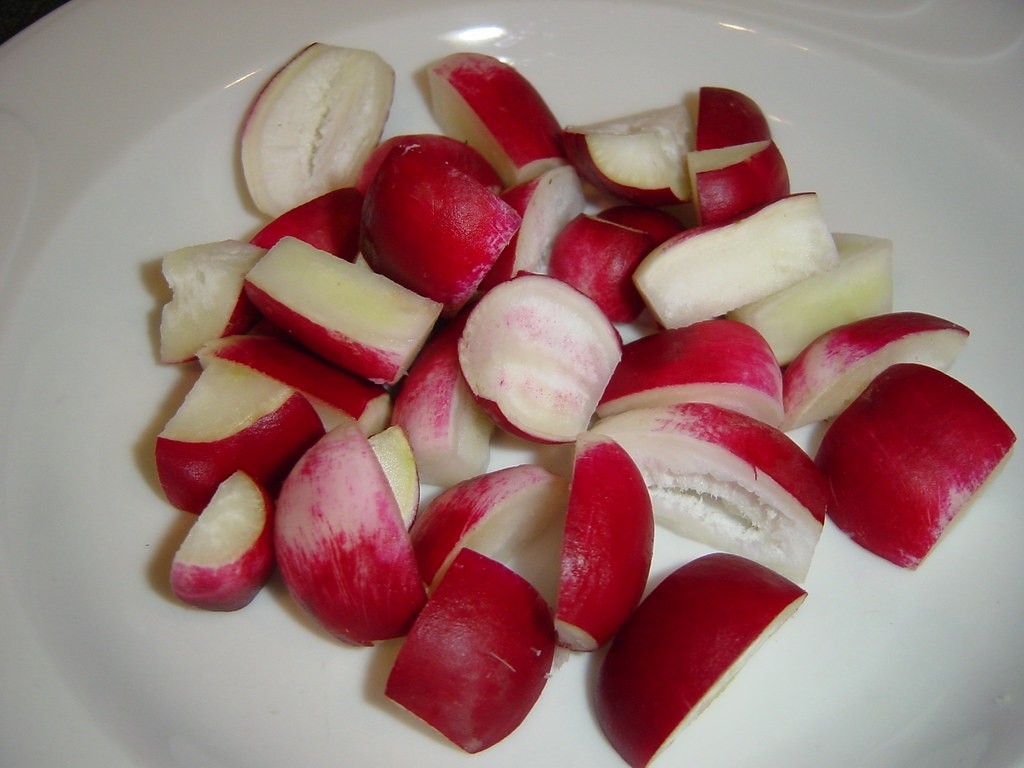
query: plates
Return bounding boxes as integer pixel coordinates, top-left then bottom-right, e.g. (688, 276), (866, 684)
(0, 0), (1024, 768)
